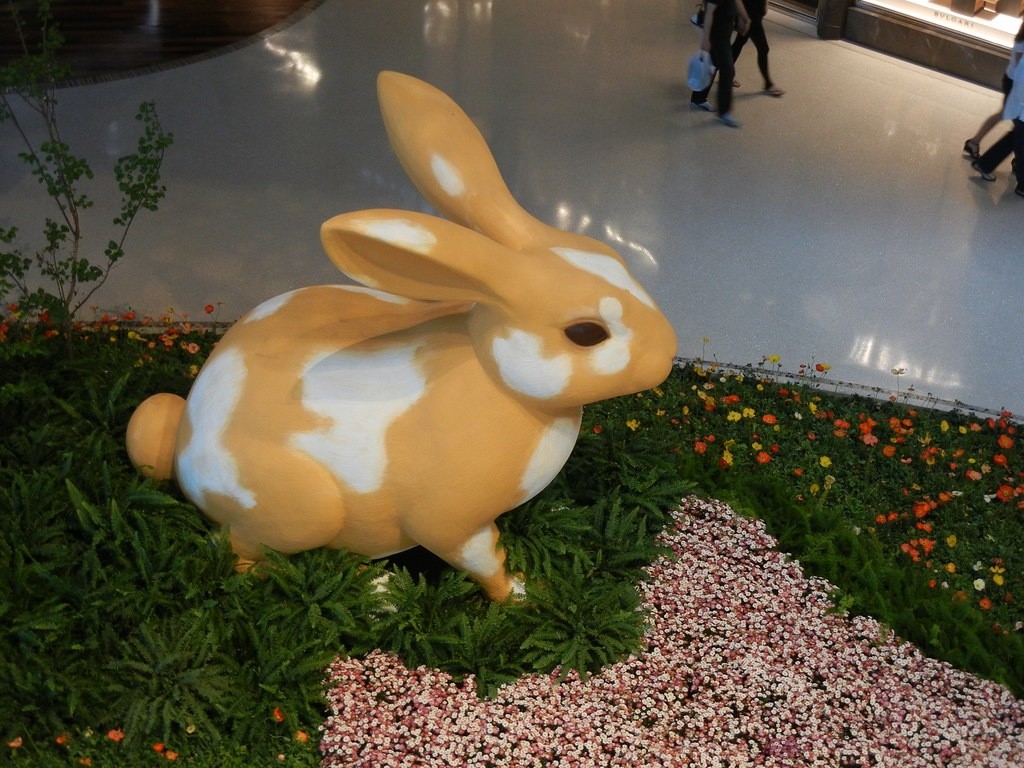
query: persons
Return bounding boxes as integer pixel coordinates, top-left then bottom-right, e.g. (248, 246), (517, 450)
(690, 0), (784, 127)
(963, 10), (1024, 197)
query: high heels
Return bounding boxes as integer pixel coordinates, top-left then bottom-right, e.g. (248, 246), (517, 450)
(964, 139), (981, 158)
(1012, 157), (1017, 172)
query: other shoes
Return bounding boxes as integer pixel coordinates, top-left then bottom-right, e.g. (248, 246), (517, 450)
(715, 77), (742, 88)
(763, 87), (785, 95)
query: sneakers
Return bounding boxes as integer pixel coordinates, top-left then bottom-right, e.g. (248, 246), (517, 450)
(690, 101), (716, 112)
(971, 160), (996, 180)
(1015, 184), (1024, 197)
(712, 113), (742, 126)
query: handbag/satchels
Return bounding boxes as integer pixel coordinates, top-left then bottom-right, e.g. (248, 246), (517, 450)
(687, 48), (715, 91)
(695, 3), (705, 25)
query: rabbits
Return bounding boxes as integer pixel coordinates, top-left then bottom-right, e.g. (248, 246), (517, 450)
(125, 70), (678, 606)
